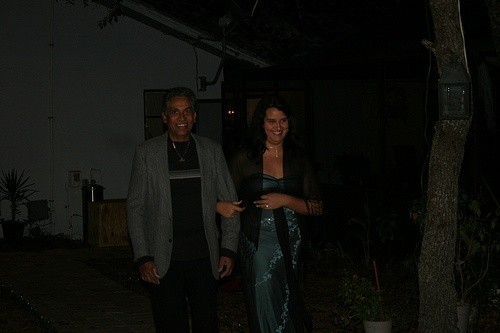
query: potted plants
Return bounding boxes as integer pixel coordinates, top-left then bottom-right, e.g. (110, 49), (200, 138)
(0, 168), (40, 243)
(334, 271), (398, 333)
(415, 191), (494, 332)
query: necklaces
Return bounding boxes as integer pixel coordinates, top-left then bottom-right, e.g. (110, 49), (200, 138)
(266, 143), (281, 158)
(170, 134), (191, 162)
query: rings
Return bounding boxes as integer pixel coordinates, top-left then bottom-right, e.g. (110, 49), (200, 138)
(265, 204), (268, 208)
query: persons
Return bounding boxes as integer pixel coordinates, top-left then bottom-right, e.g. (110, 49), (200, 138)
(216, 93), (323, 333)
(126, 87), (240, 333)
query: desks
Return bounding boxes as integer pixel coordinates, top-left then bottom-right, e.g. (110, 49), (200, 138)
(87, 200), (132, 247)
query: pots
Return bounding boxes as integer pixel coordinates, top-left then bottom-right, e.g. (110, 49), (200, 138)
(82, 180), (105, 201)
